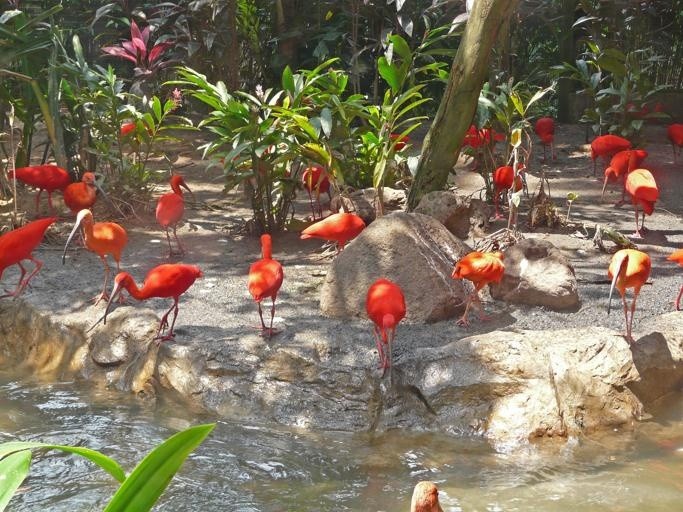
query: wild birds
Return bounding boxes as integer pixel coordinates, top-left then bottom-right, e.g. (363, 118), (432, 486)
(153, 174), (195, 259)
(0, 165), (101, 305)
(60, 209), (130, 308)
(535, 117), (558, 163)
(487, 165), (524, 222)
(117, 119), (158, 169)
(246, 233), (284, 342)
(300, 213), (366, 251)
(103, 263), (202, 343)
(664, 248), (683, 311)
(601, 150), (648, 209)
(450, 251), (505, 329)
(366, 279), (406, 371)
(300, 166), (334, 219)
(606, 248), (651, 344)
(411, 480), (444, 512)
(589, 135), (632, 175)
(623, 151), (660, 239)
